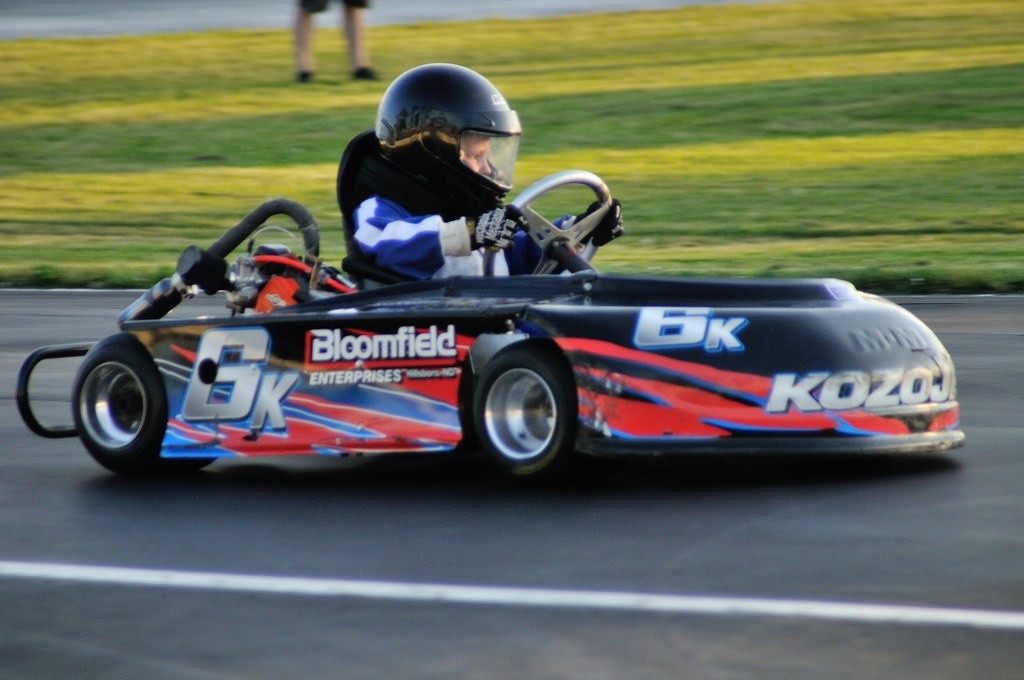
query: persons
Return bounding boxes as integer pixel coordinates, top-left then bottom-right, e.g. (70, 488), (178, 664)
(297, 0), (380, 83)
(334, 64), (624, 292)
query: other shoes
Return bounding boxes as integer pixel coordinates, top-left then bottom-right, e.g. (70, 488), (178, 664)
(297, 71), (311, 82)
(354, 67), (377, 81)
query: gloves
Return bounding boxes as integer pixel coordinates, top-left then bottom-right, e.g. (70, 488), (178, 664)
(573, 198), (624, 246)
(466, 204), (530, 248)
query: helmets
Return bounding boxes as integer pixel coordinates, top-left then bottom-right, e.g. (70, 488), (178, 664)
(373, 62), (522, 214)
